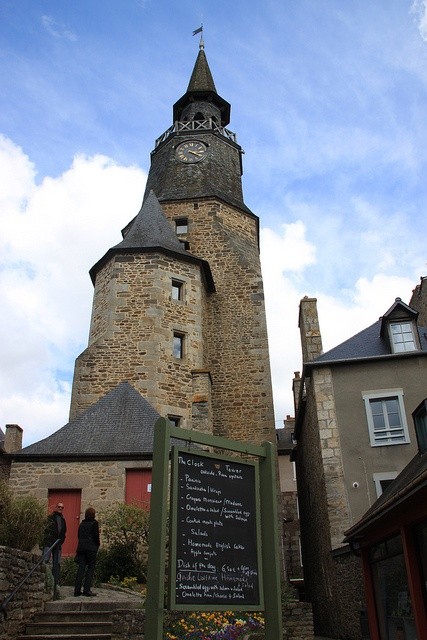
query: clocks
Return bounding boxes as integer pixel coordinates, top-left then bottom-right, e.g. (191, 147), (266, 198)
(175, 139), (209, 165)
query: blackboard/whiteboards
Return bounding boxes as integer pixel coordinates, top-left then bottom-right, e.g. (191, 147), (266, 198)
(175, 451), (259, 605)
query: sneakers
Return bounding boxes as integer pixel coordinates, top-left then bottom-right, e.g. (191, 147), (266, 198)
(74, 592), (81, 596)
(83, 590), (96, 596)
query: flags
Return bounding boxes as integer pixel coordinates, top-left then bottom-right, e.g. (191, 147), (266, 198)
(191, 26), (203, 36)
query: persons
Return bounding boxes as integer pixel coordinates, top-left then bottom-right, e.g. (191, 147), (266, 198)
(73, 507), (100, 596)
(42, 503), (66, 587)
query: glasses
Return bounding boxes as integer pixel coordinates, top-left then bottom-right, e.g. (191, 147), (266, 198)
(57, 506), (64, 509)
